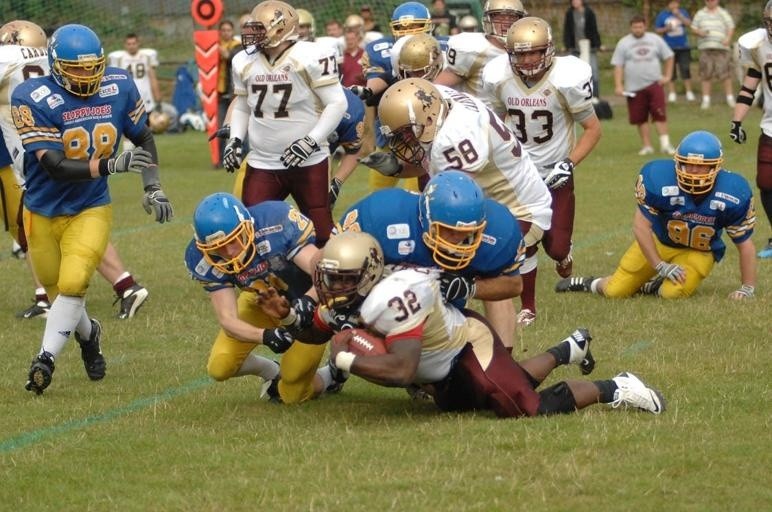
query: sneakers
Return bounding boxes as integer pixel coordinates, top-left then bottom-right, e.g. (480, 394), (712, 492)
(725, 95), (736, 108)
(73, 319), (108, 381)
(15, 300), (52, 319)
(24, 347), (56, 398)
(658, 143), (675, 155)
(685, 90), (696, 101)
(667, 91), (677, 103)
(638, 277), (665, 296)
(607, 371), (667, 416)
(8, 242), (26, 259)
(325, 358), (350, 396)
(554, 275), (593, 294)
(555, 252), (574, 278)
(756, 238), (772, 259)
(516, 309), (536, 326)
(700, 95), (712, 110)
(560, 328), (596, 375)
(407, 383), (435, 403)
(638, 145), (656, 156)
(112, 281), (149, 320)
(257, 360), (284, 406)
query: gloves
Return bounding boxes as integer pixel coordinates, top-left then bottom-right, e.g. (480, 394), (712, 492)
(437, 271), (477, 305)
(655, 261), (684, 281)
(540, 158), (575, 193)
(728, 120), (747, 144)
(346, 85), (374, 101)
(262, 326), (294, 355)
(280, 136), (319, 171)
(291, 295), (316, 327)
(141, 183), (175, 224)
(734, 285), (755, 297)
(222, 137), (242, 173)
(207, 124), (230, 142)
(329, 176), (343, 210)
(356, 151), (404, 178)
(98, 146), (153, 175)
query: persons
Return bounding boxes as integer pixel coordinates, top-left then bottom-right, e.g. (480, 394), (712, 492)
(436, 0), (528, 97)
(360, 5), (379, 32)
(325, 20), (345, 48)
(563, 0), (601, 106)
(218, 20), (241, 131)
(690, 0), (736, 111)
(344, 14), (382, 56)
(205, 83), (366, 210)
(330, 169), (523, 308)
(227, 13), (253, 87)
(1, 134), (25, 264)
(185, 192), (348, 406)
(8, 22), (173, 396)
(356, 75), (553, 355)
(0, 18), (148, 321)
(106, 33), (162, 128)
(219, 0), (348, 247)
(296, 9), (344, 80)
(654, 0), (696, 103)
(431, 0), (458, 35)
(252, 230), (668, 419)
(348, 2), (449, 192)
(728, 0), (772, 258)
(478, 15), (602, 326)
(387, 34), (470, 92)
(553, 130), (757, 303)
(611, 16), (677, 156)
(341, 29), (365, 86)
(328, 84), (366, 155)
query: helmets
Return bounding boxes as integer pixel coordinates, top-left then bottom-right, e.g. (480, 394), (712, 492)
(504, 17), (554, 81)
(313, 232), (384, 313)
(378, 78), (449, 166)
(398, 32), (443, 80)
(343, 14), (364, 29)
(672, 131), (724, 195)
(47, 24), (106, 97)
(148, 110), (171, 133)
(241, 0), (301, 56)
(389, 2), (433, 42)
(0, 20), (47, 49)
(419, 170), (487, 271)
(391, 35), (414, 77)
(189, 192), (257, 275)
(481, 0), (528, 43)
(294, 9), (317, 42)
(460, 15), (482, 33)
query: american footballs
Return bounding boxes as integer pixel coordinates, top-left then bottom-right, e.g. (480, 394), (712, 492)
(334, 328), (386, 359)
